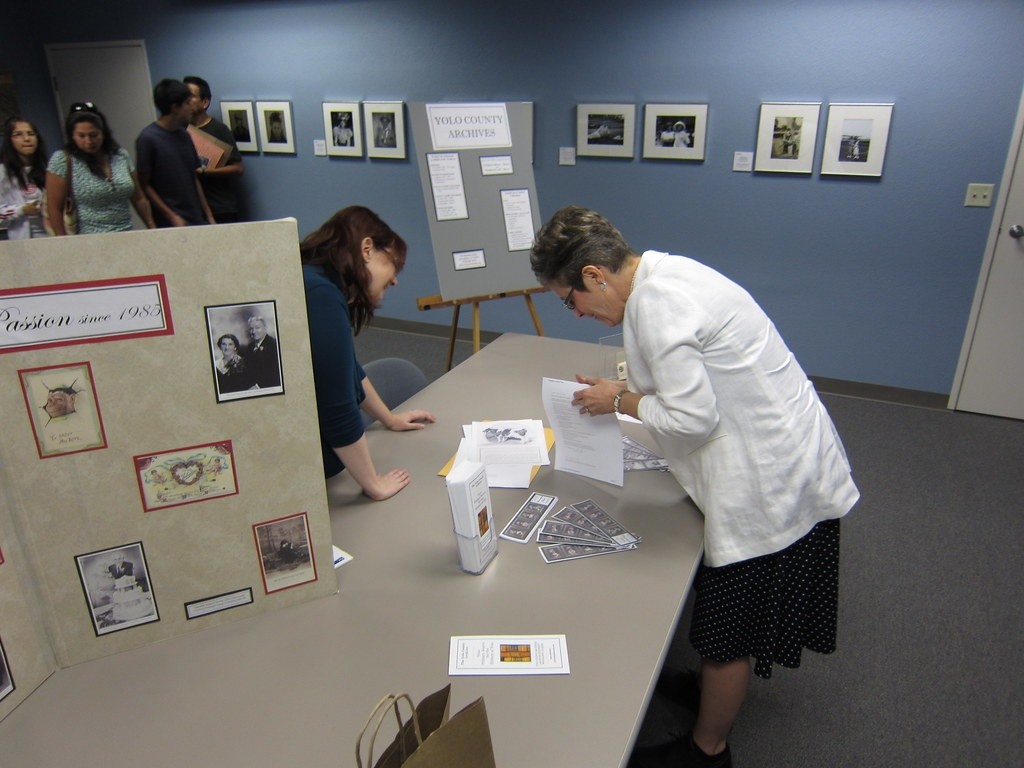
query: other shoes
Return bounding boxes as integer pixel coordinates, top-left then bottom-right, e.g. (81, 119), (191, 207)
(655, 667), (701, 714)
(629, 730), (732, 768)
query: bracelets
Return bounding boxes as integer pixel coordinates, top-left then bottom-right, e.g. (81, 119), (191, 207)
(613, 390), (630, 414)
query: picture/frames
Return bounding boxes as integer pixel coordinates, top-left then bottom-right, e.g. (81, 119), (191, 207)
(362, 100), (409, 161)
(753, 102), (822, 175)
(256, 100), (297, 156)
(219, 100), (260, 154)
(320, 100), (366, 159)
(820, 102), (895, 179)
(641, 103), (709, 162)
(575, 102), (638, 160)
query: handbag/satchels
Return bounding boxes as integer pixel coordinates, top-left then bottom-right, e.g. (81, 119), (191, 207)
(39, 185), (77, 236)
(355, 682), (497, 768)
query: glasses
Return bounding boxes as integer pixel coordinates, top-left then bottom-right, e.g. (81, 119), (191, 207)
(70, 100), (100, 112)
(377, 242), (403, 272)
(562, 264), (600, 310)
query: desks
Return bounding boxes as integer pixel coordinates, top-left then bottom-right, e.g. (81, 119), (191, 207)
(0, 333), (707, 768)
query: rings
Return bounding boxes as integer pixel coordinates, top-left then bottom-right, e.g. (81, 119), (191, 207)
(585, 407), (588, 412)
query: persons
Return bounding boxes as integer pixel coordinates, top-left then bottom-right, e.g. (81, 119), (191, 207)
(598, 121), (609, 136)
(846, 136), (862, 161)
(662, 121), (690, 147)
(108, 553), (133, 579)
(214, 316), (281, 394)
(136, 78), (216, 229)
(45, 102), (157, 236)
(268, 112), (287, 143)
(183, 75), (244, 224)
(782, 128), (798, 155)
(233, 113), (250, 142)
(333, 116), (353, 146)
(376, 116), (396, 147)
(0, 115), (49, 240)
(530, 207), (860, 768)
(300, 206), (436, 501)
